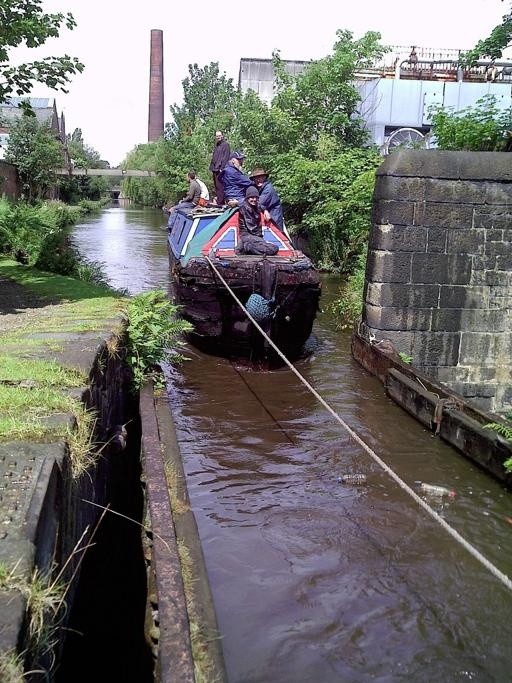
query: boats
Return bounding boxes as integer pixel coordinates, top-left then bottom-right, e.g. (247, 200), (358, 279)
(164, 197), (323, 369)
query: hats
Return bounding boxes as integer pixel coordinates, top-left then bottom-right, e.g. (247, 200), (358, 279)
(244, 186), (260, 200)
(230, 152), (246, 159)
(248, 168), (269, 180)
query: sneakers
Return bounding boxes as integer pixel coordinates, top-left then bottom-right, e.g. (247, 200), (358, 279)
(160, 225), (171, 232)
(234, 243), (243, 255)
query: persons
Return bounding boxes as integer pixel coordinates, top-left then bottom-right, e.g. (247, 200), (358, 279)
(209, 131), (231, 205)
(218, 151), (255, 208)
(228, 168), (283, 230)
(235, 186), (279, 255)
(195, 176), (209, 206)
(160, 172), (201, 234)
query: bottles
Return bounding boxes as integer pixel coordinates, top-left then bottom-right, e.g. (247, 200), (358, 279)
(337, 474), (366, 485)
(420, 482), (456, 498)
